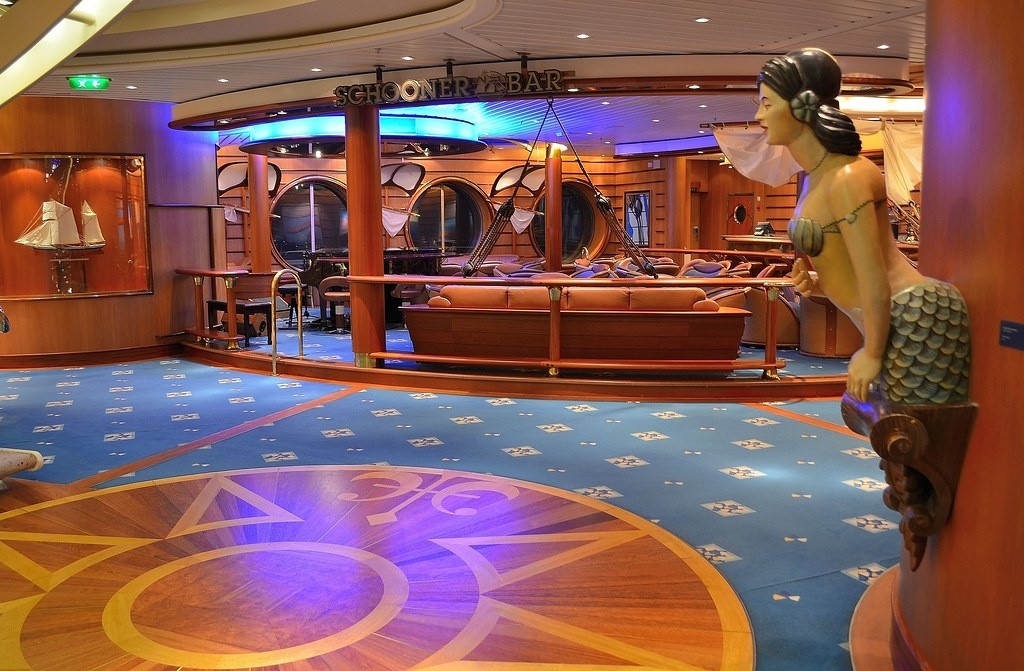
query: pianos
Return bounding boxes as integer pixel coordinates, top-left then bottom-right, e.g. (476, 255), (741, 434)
(297, 247), (444, 334)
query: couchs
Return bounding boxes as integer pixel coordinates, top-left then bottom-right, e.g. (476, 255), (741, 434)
(396, 260), (864, 377)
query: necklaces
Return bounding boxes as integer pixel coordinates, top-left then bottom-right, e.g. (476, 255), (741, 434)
(805, 148), (827, 176)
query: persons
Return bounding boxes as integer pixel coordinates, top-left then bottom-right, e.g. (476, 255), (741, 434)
(753, 44), (969, 406)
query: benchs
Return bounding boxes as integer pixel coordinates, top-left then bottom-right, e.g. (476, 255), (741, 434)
(278, 284), (308, 329)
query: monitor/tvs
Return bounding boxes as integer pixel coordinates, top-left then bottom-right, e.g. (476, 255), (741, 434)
(758, 221), (776, 234)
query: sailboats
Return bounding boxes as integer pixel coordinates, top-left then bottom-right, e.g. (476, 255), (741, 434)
(14, 197), (106, 249)
